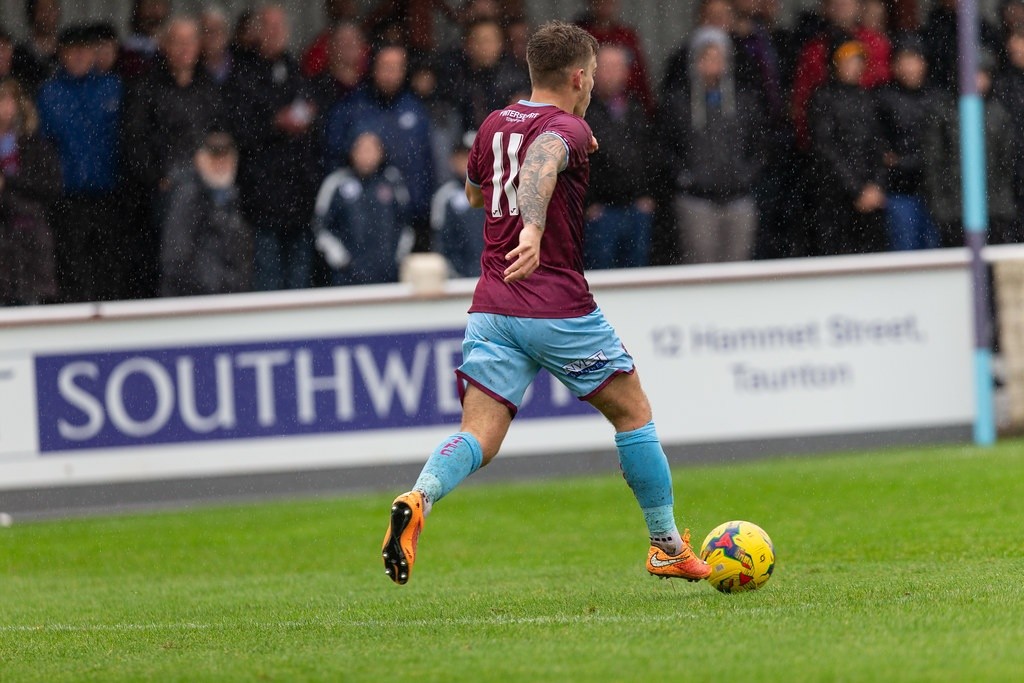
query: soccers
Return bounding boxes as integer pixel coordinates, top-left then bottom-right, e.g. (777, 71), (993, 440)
(699, 520), (776, 595)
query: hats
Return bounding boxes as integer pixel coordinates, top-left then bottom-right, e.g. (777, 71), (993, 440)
(824, 24), (867, 69)
(684, 23), (739, 133)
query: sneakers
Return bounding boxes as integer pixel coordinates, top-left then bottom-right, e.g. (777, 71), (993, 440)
(380, 490), (426, 586)
(646, 527), (712, 583)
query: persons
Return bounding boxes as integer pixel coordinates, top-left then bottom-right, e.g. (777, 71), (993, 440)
(381, 17), (712, 585)
(0, 0), (1024, 308)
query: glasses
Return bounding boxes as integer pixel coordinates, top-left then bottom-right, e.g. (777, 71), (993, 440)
(203, 138), (236, 158)
